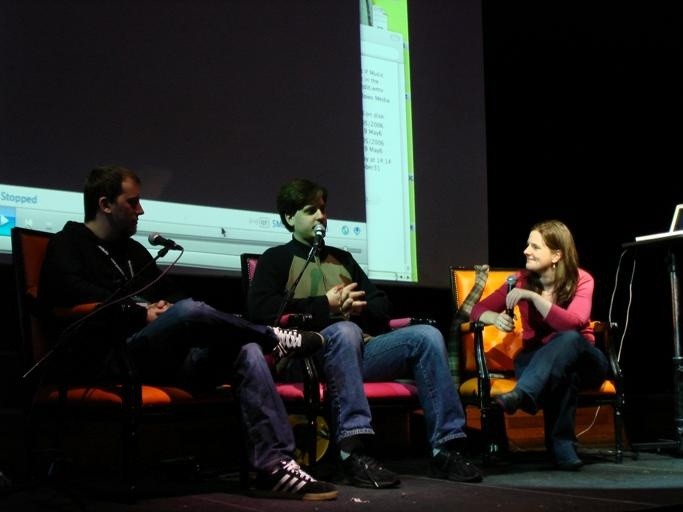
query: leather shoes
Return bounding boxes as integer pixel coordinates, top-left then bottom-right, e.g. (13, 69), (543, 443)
(559, 459), (582, 470)
(494, 390), (519, 415)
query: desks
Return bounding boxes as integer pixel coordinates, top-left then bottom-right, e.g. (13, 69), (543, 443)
(622, 235), (682, 361)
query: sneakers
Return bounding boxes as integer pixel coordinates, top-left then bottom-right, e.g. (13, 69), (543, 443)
(343, 454), (400, 488)
(266, 326), (323, 357)
(249, 460), (338, 501)
(426, 449), (482, 482)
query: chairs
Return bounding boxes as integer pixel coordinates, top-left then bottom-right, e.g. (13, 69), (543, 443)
(10, 225), (235, 511)
(240, 252), (442, 481)
(450, 267), (624, 475)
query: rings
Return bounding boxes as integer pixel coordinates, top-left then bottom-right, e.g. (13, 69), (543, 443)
(499, 321), (503, 327)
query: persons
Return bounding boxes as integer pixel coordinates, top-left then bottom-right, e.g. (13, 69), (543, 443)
(248, 179), (482, 488)
(470, 219), (611, 471)
(36, 165), (338, 501)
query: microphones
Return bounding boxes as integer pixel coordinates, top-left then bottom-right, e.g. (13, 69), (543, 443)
(313, 223), (326, 257)
(507, 274), (518, 319)
(148, 232), (183, 250)
